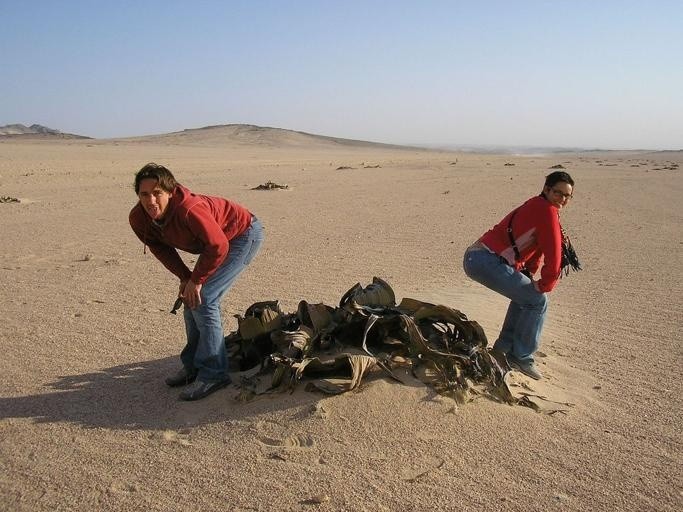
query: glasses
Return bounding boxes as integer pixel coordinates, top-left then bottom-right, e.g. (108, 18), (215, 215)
(549, 186), (573, 199)
(170, 297), (183, 316)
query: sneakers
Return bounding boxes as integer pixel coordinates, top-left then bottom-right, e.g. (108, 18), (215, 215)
(179, 376), (233, 400)
(493, 345), (543, 380)
(165, 365), (200, 387)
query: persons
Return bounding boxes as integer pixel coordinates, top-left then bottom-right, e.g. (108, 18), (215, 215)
(129, 163), (264, 399)
(463, 172), (582, 381)
